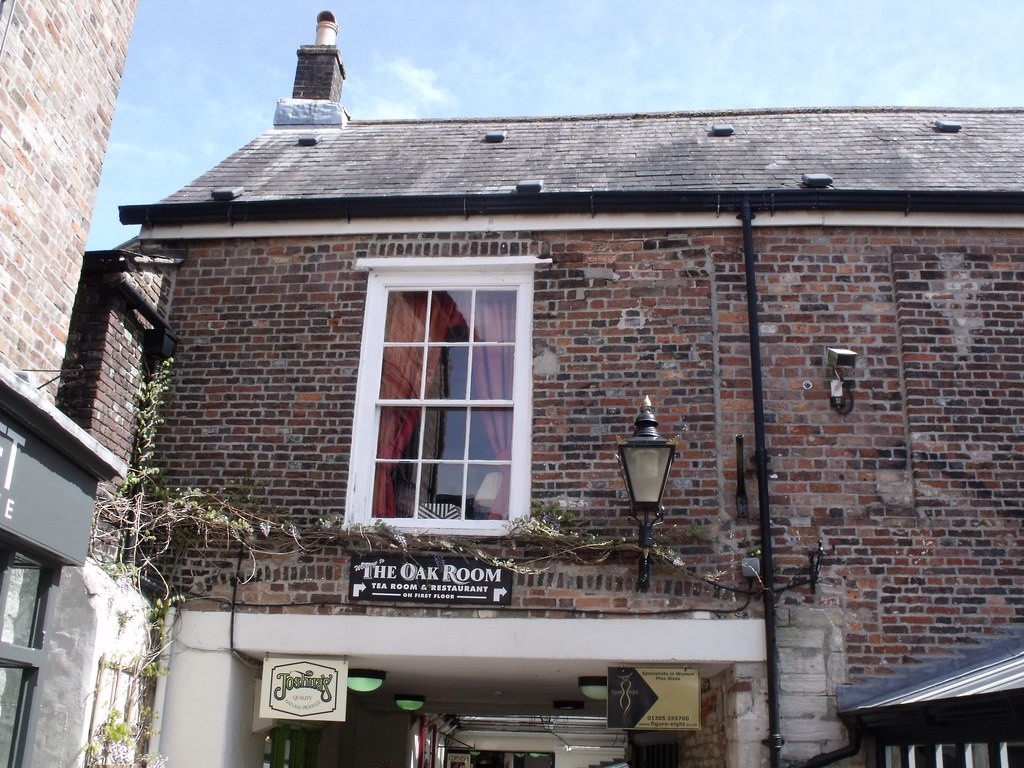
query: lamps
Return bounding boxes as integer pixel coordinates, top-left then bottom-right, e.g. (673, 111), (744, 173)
(473, 471), (503, 508)
(395, 693), (426, 711)
(578, 675), (609, 700)
(346, 667), (387, 692)
(617, 395), (676, 593)
(561, 745), (625, 756)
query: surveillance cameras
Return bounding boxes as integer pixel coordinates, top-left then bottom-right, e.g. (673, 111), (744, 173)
(826, 348), (857, 370)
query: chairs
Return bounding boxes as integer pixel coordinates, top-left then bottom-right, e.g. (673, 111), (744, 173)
(416, 502), (467, 520)
